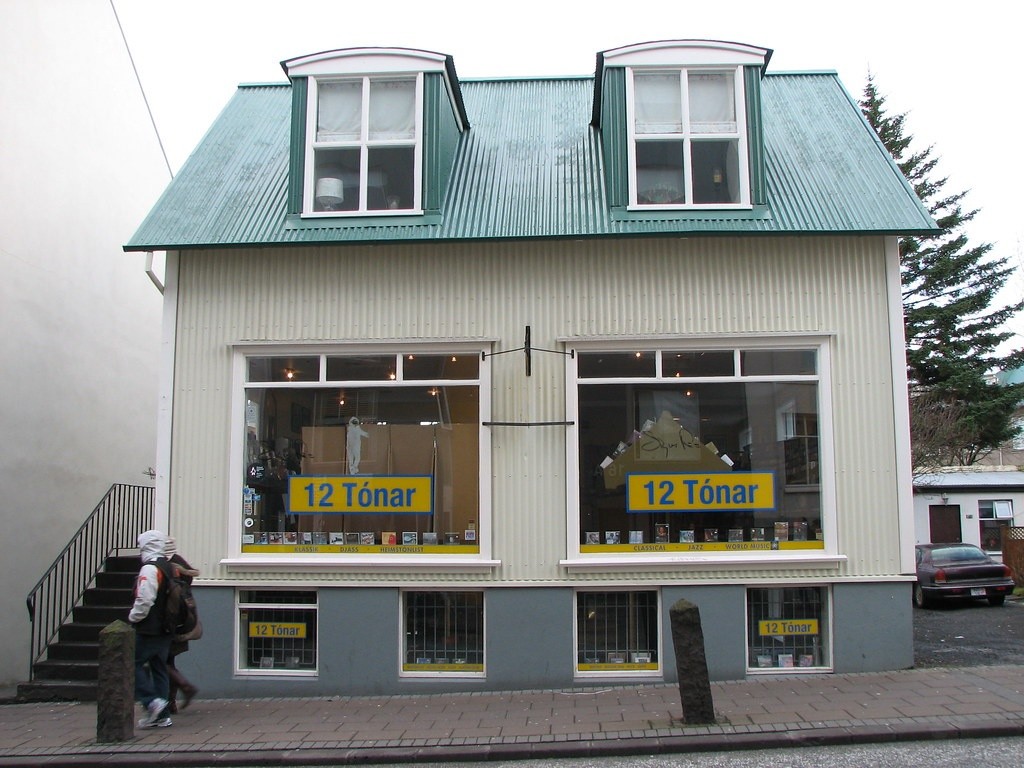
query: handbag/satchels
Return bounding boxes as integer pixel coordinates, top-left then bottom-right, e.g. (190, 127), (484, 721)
(177, 578), (202, 641)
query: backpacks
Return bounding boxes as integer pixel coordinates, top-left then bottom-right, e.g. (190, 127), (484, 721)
(149, 559), (181, 626)
(177, 579), (198, 633)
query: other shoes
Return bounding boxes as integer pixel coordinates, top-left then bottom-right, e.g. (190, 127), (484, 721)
(168, 702), (179, 716)
(181, 684), (198, 710)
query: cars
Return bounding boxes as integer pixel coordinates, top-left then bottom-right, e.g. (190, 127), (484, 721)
(913, 543), (1015, 608)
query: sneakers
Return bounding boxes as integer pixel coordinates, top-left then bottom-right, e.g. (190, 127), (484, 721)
(147, 698), (170, 725)
(138, 715), (172, 728)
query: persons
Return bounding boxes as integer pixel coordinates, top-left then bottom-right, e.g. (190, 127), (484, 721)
(127, 530), (199, 728)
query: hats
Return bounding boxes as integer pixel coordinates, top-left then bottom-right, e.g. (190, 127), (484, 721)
(164, 540), (177, 554)
